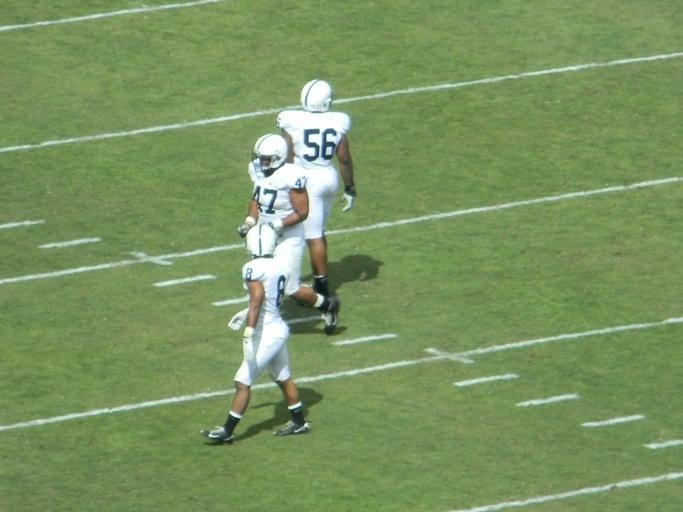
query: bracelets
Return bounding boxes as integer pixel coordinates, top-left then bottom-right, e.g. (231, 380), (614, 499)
(242, 326), (254, 338)
(273, 219), (283, 231)
(345, 184), (355, 193)
(246, 216), (256, 226)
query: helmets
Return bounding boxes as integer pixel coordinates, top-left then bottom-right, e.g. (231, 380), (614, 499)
(300, 79), (332, 112)
(246, 223), (275, 257)
(252, 134), (288, 173)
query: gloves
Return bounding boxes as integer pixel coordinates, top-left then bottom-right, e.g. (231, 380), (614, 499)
(228, 311), (246, 331)
(238, 224), (249, 237)
(341, 189), (356, 212)
(243, 336), (254, 362)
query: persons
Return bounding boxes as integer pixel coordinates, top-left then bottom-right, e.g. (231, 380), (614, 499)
(237, 134), (340, 335)
(276, 79), (356, 307)
(199, 223), (309, 444)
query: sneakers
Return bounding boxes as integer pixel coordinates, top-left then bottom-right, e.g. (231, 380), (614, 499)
(200, 426), (233, 442)
(274, 421), (309, 436)
(324, 298), (339, 334)
